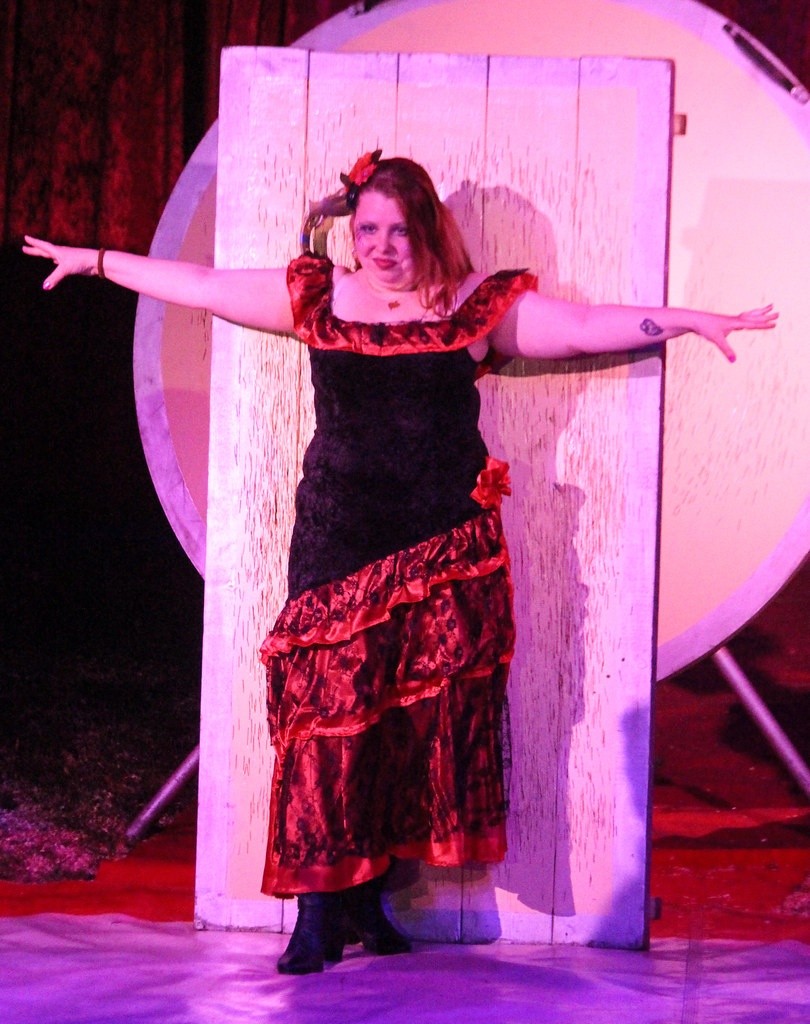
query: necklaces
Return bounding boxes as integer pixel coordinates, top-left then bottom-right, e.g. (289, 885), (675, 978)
(366, 279), (409, 310)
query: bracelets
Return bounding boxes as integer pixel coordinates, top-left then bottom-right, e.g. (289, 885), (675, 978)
(96, 244), (106, 280)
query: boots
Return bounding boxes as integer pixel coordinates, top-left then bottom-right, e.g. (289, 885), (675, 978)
(275, 891), (345, 974)
(344, 880), (413, 956)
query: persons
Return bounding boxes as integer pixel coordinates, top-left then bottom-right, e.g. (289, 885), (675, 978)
(22, 151), (778, 975)
